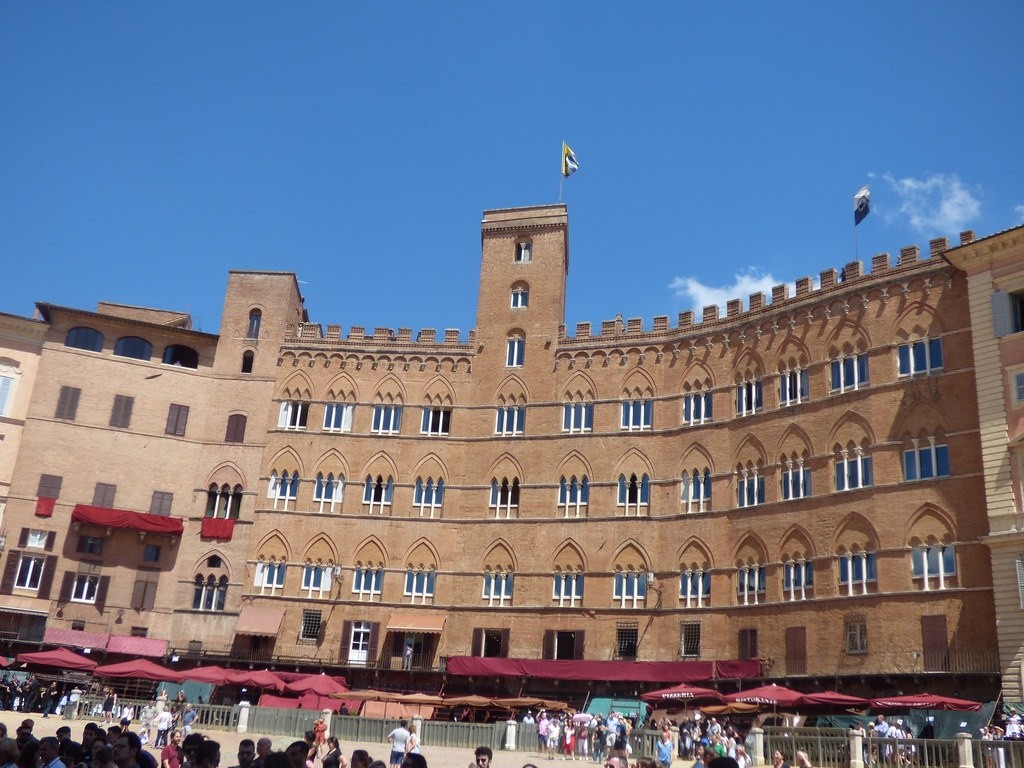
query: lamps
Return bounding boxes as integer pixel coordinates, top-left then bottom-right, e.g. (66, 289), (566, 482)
(59, 603), (65, 609)
(117, 608), (125, 616)
(333, 564), (343, 586)
(646, 570), (662, 601)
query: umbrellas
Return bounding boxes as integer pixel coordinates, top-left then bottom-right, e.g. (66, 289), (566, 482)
(723, 685), (870, 727)
(701, 702), (761, 714)
(327, 689), (578, 719)
(641, 683), (724, 716)
(181, 665), (351, 711)
(869, 691), (984, 724)
(17, 646), (187, 700)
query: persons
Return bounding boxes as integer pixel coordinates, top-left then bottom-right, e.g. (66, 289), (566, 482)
(468, 707), (1024, 768)
(0, 673), (430, 768)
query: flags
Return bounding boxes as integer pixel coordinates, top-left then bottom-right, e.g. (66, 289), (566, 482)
(562, 143), (579, 177)
(853, 185), (870, 226)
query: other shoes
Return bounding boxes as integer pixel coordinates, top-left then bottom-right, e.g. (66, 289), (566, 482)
(549, 755), (602, 763)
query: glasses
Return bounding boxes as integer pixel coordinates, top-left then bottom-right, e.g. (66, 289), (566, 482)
(477, 758), (489, 762)
(604, 764), (614, 768)
(21, 733), (30, 737)
(185, 748), (197, 754)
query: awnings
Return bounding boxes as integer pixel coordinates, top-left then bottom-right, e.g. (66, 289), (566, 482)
(233, 604), (287, 638)
(43, 626), (169, 657)
(385, 613), (446, 634)
(446, 656), (762, 681)
